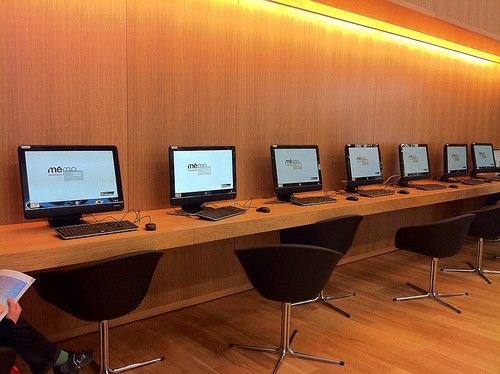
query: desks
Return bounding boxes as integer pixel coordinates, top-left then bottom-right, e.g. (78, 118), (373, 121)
(0, 172), (500, 274)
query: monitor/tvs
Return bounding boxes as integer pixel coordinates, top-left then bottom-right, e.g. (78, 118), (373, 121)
(440, 144), (469, 182)
(345, 144), (384, 196)
(493, 149), (500, 176)
(471, 143), (496, 178)
(270, 144), (323, 201)
(18, 144), (124, 227)
(168, 146), (237, 215)
(397, 144), (430, 187)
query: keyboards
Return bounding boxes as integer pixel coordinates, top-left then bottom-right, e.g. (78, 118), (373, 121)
(359, 188), (395, 197)
(483, 176), (500, 181)
(291, 196), (337, 206)
(53, 219), (139, 240)
(195, 205), (246, 220)
(414, 184), (446, 191)
(462, 179), (484, 185)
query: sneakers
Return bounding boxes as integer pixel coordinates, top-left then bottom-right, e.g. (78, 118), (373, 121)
(53, 348), (97, 374)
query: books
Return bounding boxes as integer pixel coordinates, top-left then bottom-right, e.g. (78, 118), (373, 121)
(0, 269), (36, 324)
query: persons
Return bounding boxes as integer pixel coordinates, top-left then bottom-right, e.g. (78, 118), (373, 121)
(0, 296), (96, 374)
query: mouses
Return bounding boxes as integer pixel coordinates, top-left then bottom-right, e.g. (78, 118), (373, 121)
(256, 206), (270, 213)
(398, 189), (409, 194)
(145, 223), (156, 230)
(448, 184), (459, 188)
(346, 196), (358, 200)
(484, 179), (491, 182)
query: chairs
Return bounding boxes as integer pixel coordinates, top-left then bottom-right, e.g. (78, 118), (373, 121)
(227, 242), (345, 374)
(278, 213), (363, 318)
(392, 192), (500, 314)
(30, 252), (165, 374)
